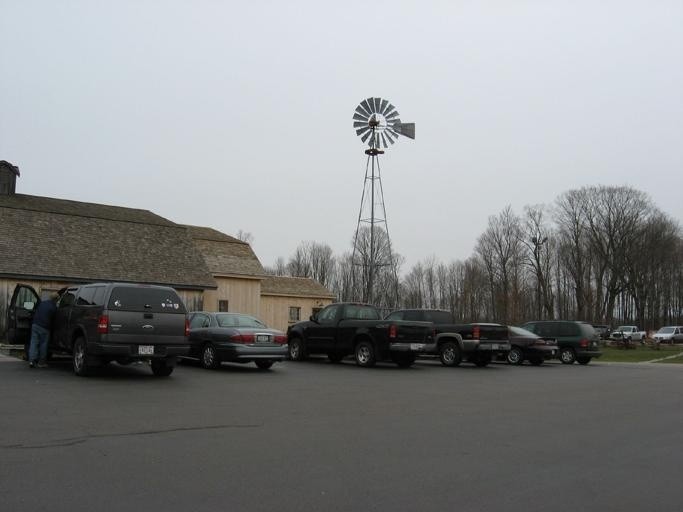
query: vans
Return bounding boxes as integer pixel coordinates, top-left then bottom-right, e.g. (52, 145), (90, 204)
(8, 282), (191, 376)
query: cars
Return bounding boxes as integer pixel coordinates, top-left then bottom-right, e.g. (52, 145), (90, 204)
(591, 324), (683, 347)
(177, 311), (290, 370)
(519, 320), (602, 365)
(469, 322), (559, 367)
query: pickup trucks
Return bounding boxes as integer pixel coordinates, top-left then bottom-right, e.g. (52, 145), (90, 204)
(382, 308), (511, 369)
(286, 301), (436, 369)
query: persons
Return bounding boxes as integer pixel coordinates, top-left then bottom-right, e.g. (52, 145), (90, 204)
(28, 291), (61, 368)
